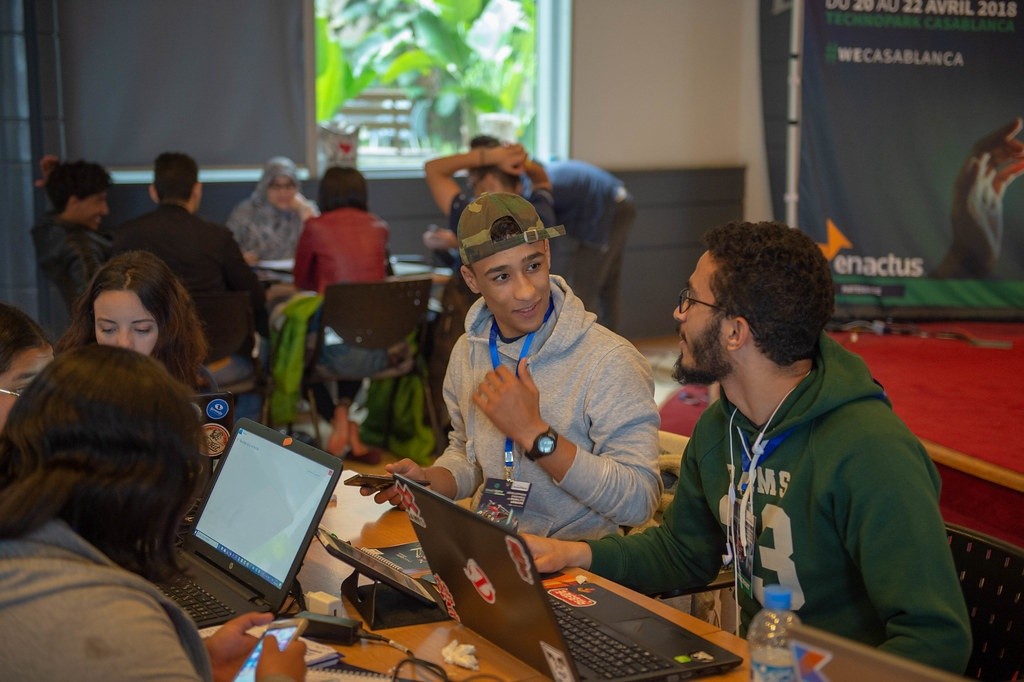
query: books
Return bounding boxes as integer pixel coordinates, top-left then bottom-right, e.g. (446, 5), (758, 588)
(361, 541), (431, 573)
(195, 622), (397, 682)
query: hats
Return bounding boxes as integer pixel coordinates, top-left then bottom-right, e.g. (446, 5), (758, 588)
(455, 190), (566, 267)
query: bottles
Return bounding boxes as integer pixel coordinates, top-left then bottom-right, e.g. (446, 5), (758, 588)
(747, 586), (802, 682)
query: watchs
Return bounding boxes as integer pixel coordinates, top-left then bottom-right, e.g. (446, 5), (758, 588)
(525, 426), (558, 462)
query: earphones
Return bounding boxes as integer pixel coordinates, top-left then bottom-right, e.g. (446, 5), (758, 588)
(752, 433), (764, 455)
(723, 543), (732, 565)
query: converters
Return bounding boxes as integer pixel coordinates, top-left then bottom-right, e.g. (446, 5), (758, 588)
(294, 611), (363, 644)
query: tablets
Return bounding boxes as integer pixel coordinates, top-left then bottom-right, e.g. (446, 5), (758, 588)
(317, 528), (438, 606)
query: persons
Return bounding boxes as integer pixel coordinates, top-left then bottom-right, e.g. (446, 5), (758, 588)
(114, 152), (268, 422)
(33, 155), (114, 310)
(360, 191), (661, 540)
(0, 302), (53, 433)
(225, 156), (319, 267)
(524, 221), (974, 676)
(296, 166), (390, 457)
(0, 345), (309, 681)
(54, 250), (210, 394)
(423, 138), (635, 330)
(929, 116), (1024, 283)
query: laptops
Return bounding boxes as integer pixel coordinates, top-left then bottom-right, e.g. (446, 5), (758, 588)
(391, 472), (742, 681)
(177, 392), (234, 525)
(152, 418), (344, 629)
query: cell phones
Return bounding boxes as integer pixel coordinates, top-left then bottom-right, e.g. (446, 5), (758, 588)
(232, 619), (307, 682)
(345, 474), (431, 491)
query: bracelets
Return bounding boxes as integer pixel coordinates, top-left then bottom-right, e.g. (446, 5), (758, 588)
(524, 159), (531, 170)
(480, 151), (486, 167)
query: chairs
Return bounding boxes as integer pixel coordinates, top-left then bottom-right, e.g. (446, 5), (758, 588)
(937, 520), (1024, 682)
(621, 428), (735, 628)
(284, 275), (449, 458)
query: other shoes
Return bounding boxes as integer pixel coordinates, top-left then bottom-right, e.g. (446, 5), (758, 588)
(346, 447), (378, 465)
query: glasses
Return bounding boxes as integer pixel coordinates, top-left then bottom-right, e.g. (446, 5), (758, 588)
(679, 288), (759, 339)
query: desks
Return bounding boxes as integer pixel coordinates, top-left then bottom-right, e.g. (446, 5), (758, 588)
(251, 253), (464, 426)
(190, 467), (804, 681)
